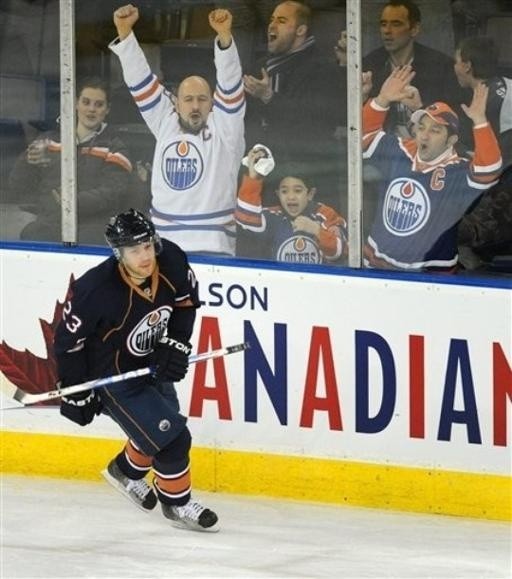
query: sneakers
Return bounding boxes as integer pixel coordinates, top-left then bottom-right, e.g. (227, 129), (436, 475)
(107, 458), (158, 511)
(162, 499), (219, 529)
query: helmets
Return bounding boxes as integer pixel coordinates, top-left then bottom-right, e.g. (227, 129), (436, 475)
(103, 208), (156, 249)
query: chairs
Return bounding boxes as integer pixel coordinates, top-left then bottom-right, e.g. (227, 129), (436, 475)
(1, 0), (512, 282)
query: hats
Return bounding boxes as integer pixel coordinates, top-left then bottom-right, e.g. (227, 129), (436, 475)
(409, 101), (460, 135)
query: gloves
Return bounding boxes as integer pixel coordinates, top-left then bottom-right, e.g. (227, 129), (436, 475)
(149, 334), (193, 384)
(59, 384), (104, 427)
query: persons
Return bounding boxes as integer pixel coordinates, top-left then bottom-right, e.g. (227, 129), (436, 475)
(334, 1), (461, 105)
(107, 4), (247, 261)
(242, 1), (326, 160)
(9, 74), (135, 245)
(232, 143), (348, 268)
(54, 206), (222, 534)
(360, 64), (503, 276)
(398, 34), (512, 154)
